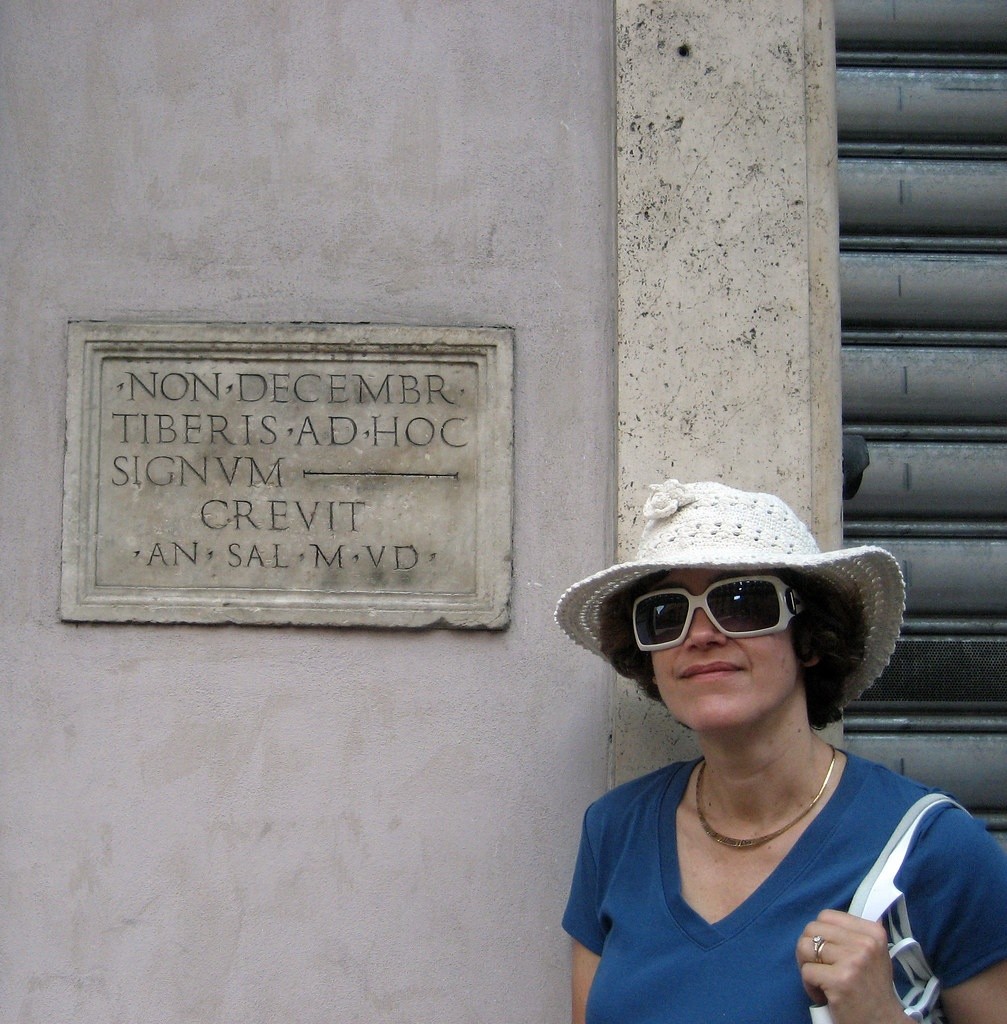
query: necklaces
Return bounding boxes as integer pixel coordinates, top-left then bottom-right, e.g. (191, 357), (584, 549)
(695, 743), (835, 849)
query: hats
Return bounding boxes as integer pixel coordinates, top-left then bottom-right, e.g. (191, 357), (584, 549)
(552, 480), (904, 713)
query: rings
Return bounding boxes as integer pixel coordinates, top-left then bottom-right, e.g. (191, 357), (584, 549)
(812, 934), (828, 964)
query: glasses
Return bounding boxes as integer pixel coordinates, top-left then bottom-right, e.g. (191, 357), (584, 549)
(632, 574), (803, 652)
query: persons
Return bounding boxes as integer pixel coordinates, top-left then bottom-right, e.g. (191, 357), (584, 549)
(551, 478), (1006, 1024)
